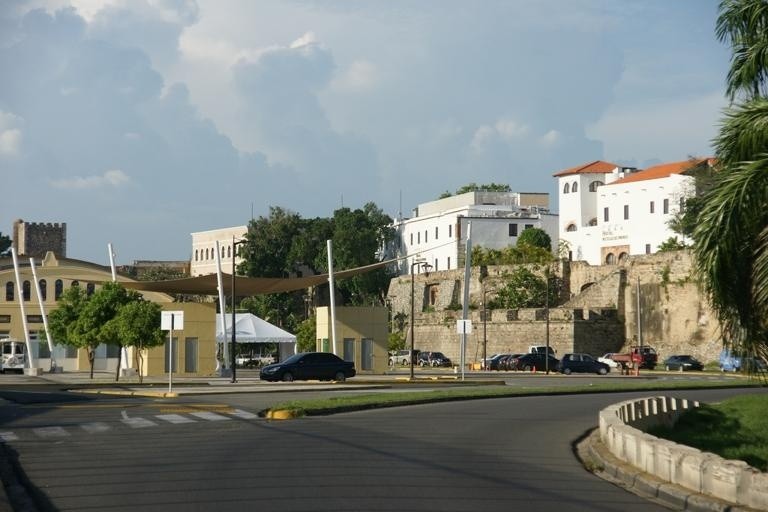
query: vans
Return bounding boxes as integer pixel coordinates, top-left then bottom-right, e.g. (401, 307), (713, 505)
(388, 348), (420, 366)
(719, 348), (760, 373)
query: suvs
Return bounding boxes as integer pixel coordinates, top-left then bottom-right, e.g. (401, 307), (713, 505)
(417, 351), (452, 368)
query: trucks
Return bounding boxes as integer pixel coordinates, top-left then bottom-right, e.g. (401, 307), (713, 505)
(611, 344), (658, 371)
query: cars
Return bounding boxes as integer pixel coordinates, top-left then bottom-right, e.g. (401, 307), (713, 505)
(479, 342), (626, 376)
(259, 352), (357, 384)
(235, 351), (280, 367)
(663, 355), (704, 371)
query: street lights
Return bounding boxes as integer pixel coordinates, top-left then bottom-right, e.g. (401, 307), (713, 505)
(410, 261), (433, 380)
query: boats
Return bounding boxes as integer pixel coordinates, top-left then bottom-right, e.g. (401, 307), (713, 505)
(481, 288), (499, 372)
